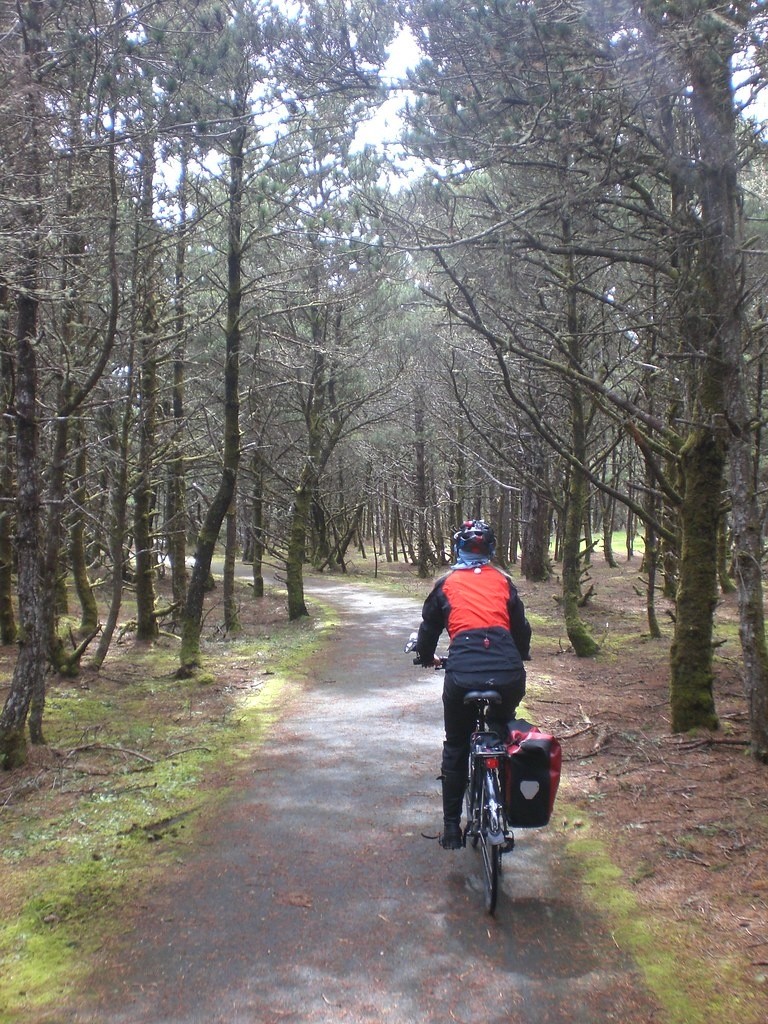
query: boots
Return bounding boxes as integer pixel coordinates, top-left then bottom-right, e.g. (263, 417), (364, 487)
(436, 768), (469, 849)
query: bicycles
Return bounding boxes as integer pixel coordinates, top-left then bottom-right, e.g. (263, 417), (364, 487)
(413, 654), (532, 916)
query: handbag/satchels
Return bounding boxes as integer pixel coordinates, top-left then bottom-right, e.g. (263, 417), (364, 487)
(502, 718), (562, 828)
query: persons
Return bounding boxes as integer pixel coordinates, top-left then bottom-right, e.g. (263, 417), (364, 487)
(416, 519), (532, 849)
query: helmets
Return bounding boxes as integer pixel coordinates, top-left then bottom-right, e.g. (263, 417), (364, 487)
(451, 519), (495, 554)
